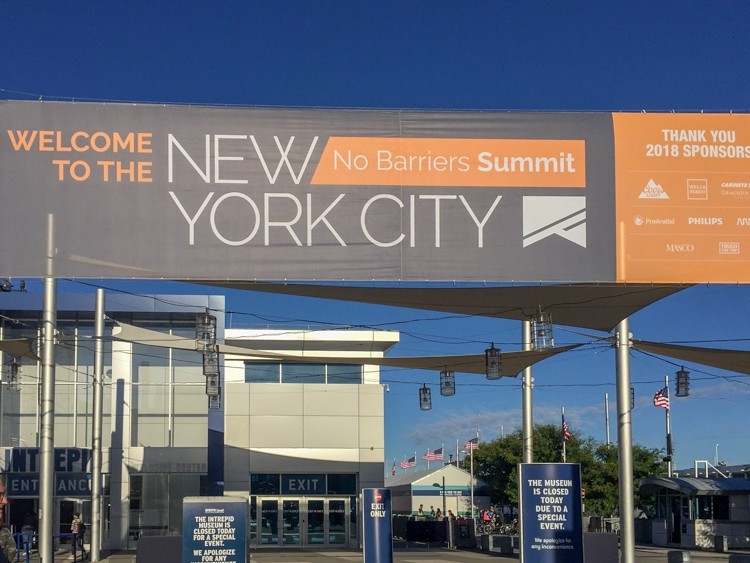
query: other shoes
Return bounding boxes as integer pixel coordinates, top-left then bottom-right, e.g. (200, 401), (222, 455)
(67, 557), (74, 560)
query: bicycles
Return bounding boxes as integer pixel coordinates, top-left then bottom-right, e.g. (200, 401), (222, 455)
(482, 520), (506, 535)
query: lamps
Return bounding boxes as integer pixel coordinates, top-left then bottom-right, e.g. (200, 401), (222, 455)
(7, 358), (23, 391)
(419, 384), (432, 411)
(196, 307), (221, 408)
(485, 343), (503, 379)
(440, 366), (455, 396)
(674, 366), (690, 397)
(530, 305), (554, 351)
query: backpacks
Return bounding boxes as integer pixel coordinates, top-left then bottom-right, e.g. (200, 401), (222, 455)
(74, 519), (86, 536)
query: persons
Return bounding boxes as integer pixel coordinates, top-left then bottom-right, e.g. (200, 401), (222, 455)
(489, 504), (519, 529)
(430, 506), (441, 518)
(448, 510), (454, 517)
(418, 503), (424, 516)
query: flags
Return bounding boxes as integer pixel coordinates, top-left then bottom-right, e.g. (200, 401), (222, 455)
(562, 413), (571, 442)
(464, 437), (479, 450)
(422, 448), (442, 461)
(399, 457), (416, 469)
(654, 386), (669, 411)
(391, 466), (395, 476)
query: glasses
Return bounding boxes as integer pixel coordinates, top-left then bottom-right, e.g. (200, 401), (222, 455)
(0, 494), (7, 501)
